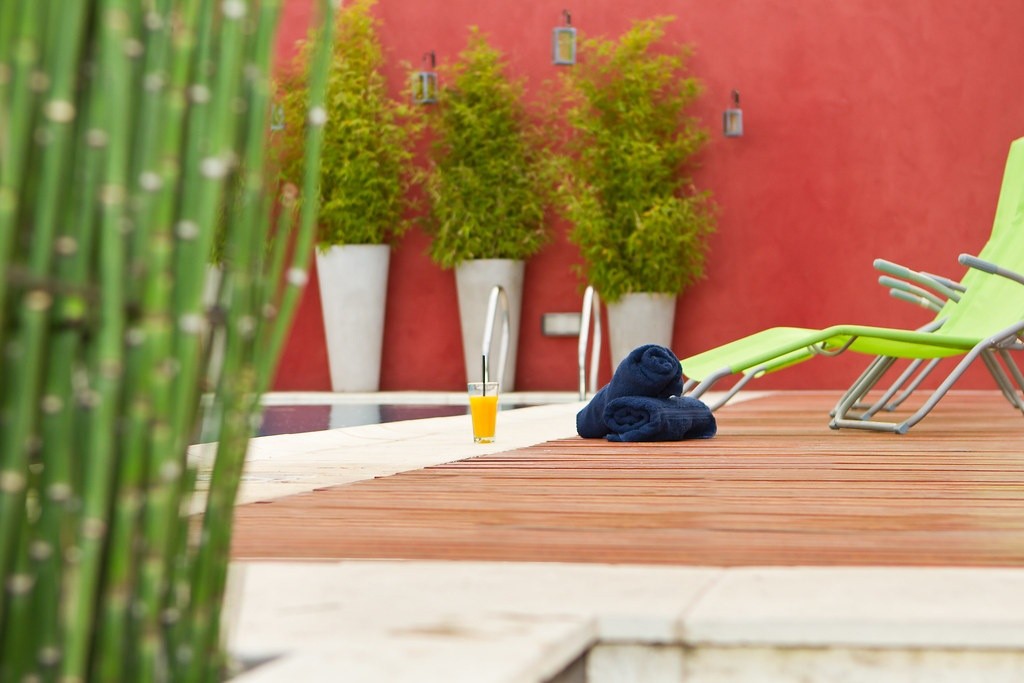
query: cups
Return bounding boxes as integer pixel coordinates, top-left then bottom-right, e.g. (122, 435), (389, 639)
(467, 382), (499, 444)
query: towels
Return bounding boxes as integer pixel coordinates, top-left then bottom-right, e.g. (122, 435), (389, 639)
(576, 345), (682, 438)
(601, 394), (717, 443)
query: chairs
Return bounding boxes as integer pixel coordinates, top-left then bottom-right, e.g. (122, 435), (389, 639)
(669, 136), (1024, 437)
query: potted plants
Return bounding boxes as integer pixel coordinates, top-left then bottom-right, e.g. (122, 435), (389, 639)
(268, 3), (417, 394)
(422, 25), (554, 395)
(540, 12), (714, 378)
